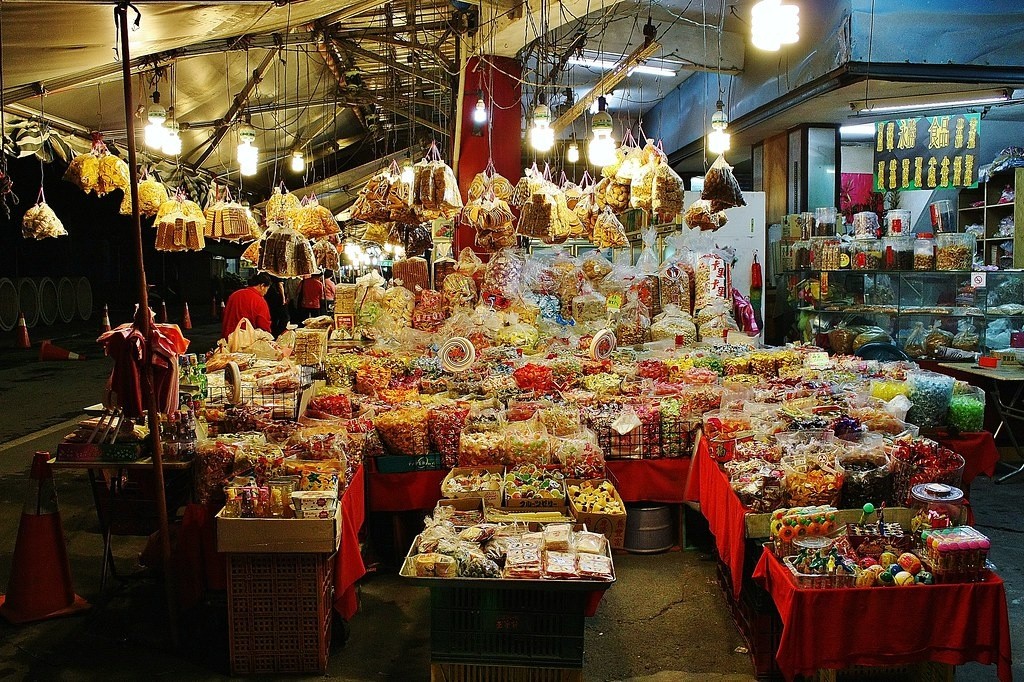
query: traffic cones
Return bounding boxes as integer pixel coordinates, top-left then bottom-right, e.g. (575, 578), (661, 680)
(12, 313), (32, 352)
(218, 298), (225, 320)
(0, 450), (92, 622)
(180, 302), (192, 329)
(158, 299), (167, 322)
(207, 297), (217, 320)
(97, 303), (111, 339)
(40, 339), (85, 360)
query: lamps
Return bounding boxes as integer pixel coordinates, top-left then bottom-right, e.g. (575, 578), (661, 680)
(850, 88), (1013, 113)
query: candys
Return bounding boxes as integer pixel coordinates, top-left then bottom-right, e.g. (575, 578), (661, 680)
(191, 320), (987, 542)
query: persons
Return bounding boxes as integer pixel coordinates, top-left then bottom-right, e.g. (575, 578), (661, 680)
(222, 273), (272, 344)
(264, 269), (335, 336)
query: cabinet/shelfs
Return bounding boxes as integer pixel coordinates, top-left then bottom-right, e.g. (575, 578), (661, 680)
(773, 269), (1024, 462)
(955, 167), (1024, 270)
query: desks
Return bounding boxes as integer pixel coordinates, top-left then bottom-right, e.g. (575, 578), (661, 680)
(752, 548), (1014, 682)
(686, 431), (974, 601)
(937, 363), (1024, 484)
(175, 464), (367, 621)
(46, 450), (195, 597)
(362, 431), (996, 556)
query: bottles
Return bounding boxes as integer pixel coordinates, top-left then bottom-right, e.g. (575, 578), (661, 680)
(911, 484), (962, 550)
(810, 199), (975, 272)
(178, 353), (207, 399)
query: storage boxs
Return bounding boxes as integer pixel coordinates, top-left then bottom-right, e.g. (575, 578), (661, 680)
(213, 507), (342, 676)
(713, 542), (953, 681)
(433, 465), (627, 549)
(426, 586), (586, 682)
